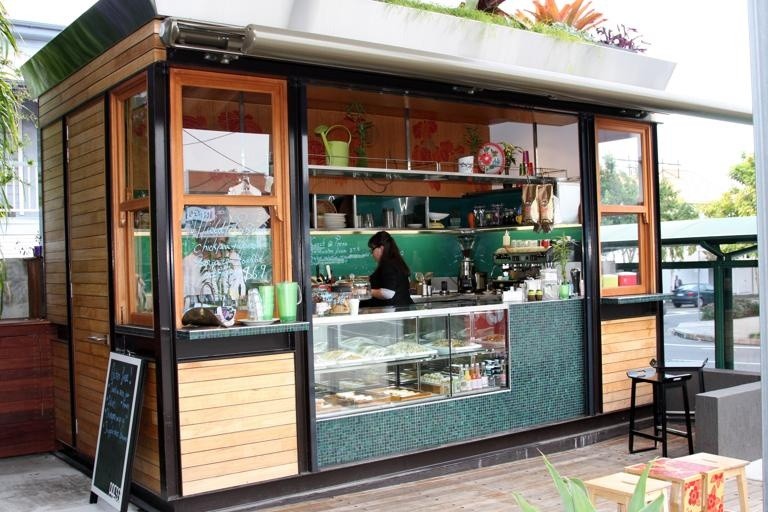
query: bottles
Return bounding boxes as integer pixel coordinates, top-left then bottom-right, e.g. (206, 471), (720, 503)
(422, 279), (428, 296)
(570, 268), (580, 295)
(428, 285), (432, 296)
(502, 229), (511, 248)
(527, 290), (535, 301)
(535, 289), (543, 301)
(527, 162), (534, 176)
(519, 163), (526, 176)
(468, 203), (516, 229)
(452, 358), (507, 394)
(523, 150), (529, 176)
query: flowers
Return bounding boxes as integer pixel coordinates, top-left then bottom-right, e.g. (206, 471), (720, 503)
(498, 141), (524, 167)
(546, 232), (580, 300)
(344, 102), (375, 153)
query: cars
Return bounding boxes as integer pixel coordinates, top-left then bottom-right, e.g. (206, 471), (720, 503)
(672, 283), (714, 308)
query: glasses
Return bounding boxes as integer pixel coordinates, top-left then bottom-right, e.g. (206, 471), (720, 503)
(371, 245), (379, 253)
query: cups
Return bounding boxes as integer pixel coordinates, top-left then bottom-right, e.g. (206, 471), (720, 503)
(395, 214), (407, 229)
(356, 213), (365, 228)
(511, 238), (557, 247)
(246, 286), (264, 321)
(450, 218), (461, 226)
(274, 281), (304, 323)
(382, 207), (394, 228)
(258, 285), (275, 321)
(349, 300), (361, 315)
(457, 155), (475, 173)
(364, 211), (374, 227)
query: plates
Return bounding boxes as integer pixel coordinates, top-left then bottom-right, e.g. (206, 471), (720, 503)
(478, 143), (506, 174)
(323, 212), (347, 228)
(407, 223), (423, 228)
(239, 318), (280, 325)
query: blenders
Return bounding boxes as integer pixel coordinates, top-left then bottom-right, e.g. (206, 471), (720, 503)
(456, 234), (480, 292)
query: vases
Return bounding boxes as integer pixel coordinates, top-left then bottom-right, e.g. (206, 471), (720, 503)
(504, 167), (511, 175)
(358, 152), (368, 167)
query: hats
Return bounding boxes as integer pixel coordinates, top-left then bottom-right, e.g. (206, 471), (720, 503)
(192, 205), (236, 230)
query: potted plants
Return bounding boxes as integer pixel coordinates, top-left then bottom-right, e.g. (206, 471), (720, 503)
(463, 127), (483, 173)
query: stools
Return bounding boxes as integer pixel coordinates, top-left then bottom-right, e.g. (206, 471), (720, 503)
(649, 358), (708, 442)
(648, 457), (725, 512)
(672, 452), (750, 512)
(584, 472), (671, 512)
(627, 367), (694, 457)
(624, 463), (702, 512)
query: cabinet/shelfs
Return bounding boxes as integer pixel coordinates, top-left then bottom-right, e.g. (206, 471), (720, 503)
(307, 302), (583, 473)
(309, 154), (558, 235)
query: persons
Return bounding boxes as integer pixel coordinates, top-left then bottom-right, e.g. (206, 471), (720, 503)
(182, 209), (246, 298)
(674, 275), (681, 288)
(360, 231), (414, 305)
